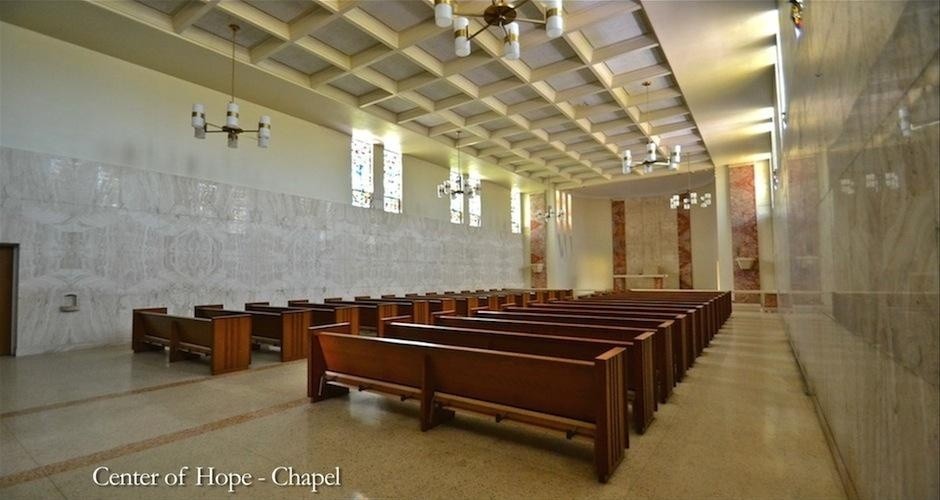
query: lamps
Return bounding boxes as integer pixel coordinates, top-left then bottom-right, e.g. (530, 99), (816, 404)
(534, 175), (567, 224)
(668, 152), (712, 212)
(436, 130), (482, 200)
(190, 23), (272, 149)
(622, 80), (681, 176)
(434, 0), (563, 62)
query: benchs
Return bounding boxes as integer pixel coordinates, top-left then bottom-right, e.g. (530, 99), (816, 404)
(131, 287), (732, 485)
